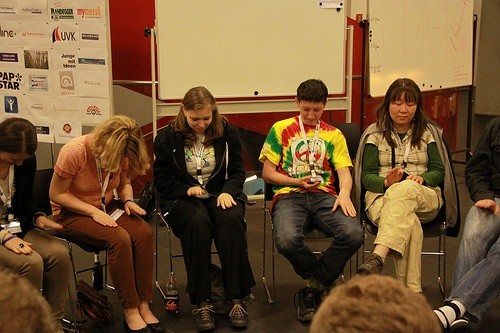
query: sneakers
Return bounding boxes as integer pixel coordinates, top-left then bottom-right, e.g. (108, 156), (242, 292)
(356, 253), (384, 277)
(319, 290), (329, 304)
(293, 287), (316, 321)
(196, 301), (215, 330)
(226, 297), (248, 327)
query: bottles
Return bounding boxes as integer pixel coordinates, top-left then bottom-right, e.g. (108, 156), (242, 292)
(165, 271), (179, 314)
(92, 252), (103, 290)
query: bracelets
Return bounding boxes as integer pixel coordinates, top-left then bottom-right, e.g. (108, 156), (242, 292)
(33, 211), (47, 226)
(124, 199), (135, 206)
(1, 235), (17, 247)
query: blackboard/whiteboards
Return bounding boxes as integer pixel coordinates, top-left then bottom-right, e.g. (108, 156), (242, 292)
(367, 0), (473, 97)
(0, 0), (113, 126)
(155, 0), (347, 100)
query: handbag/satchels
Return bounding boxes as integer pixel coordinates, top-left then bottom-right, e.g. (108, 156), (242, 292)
(76, 281), (114, 326)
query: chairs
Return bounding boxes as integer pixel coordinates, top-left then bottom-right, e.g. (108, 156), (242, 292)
(154, 187), (250, 300)
(34, 167), (114, 333)
(262, 124), (362, 303)
(363, 218), (447, 301)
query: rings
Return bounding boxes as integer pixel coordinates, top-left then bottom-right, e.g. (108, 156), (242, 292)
(19, 243), (24, 248)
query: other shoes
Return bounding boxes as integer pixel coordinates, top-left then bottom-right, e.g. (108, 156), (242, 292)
(148, 322), (159, 330)
(123, 310), (150, 333)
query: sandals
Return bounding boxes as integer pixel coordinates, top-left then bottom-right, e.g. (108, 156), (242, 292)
(428, 303), (472, 333)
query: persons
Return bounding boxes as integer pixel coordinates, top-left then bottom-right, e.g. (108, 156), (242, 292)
(432, 116), (500, 331)
(0, 265), (64, 333)
(259, 78), (363, 323)
(308, 273), (442, 333)
(0, 118), (70, 331)
(355, 77), (445, 294)
(48, 114), (166, 333)
(152, 86), (256, 329)
